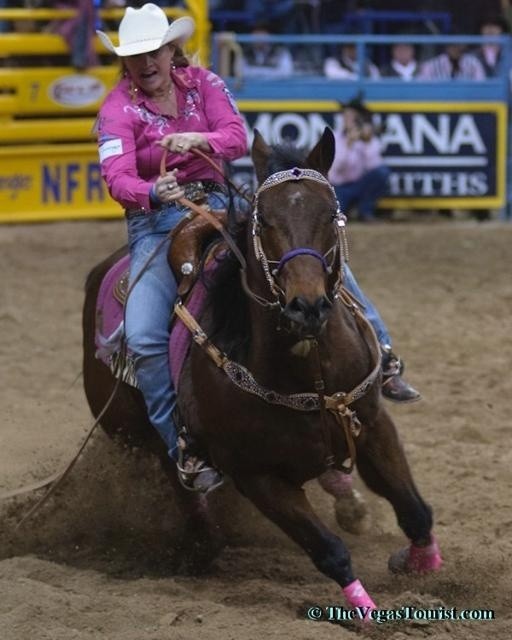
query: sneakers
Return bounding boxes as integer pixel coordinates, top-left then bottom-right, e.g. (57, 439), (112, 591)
(173, 455), (223, 493)
(381, 373), (419, 403)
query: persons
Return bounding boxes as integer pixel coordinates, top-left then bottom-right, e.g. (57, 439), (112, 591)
(322, 32), (381, 81)
(329, 95), (394, 222)
(236, 27), (297, 77)
(379, 42), (420, 80)
(95, 3), (422, 495)
(421, 42), (485, 83)
(473, 15), (508, 77)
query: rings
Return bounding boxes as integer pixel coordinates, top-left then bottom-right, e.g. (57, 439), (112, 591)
(169, 184), (174, 191)
(176, 144), (184, 148)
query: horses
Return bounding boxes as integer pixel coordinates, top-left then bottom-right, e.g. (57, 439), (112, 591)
(82, 126), (443, 632)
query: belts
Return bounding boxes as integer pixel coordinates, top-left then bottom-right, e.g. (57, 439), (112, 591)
(126, 180), (230, 217)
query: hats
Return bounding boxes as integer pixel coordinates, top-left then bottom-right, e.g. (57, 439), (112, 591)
(95, 3), (194, 57)
